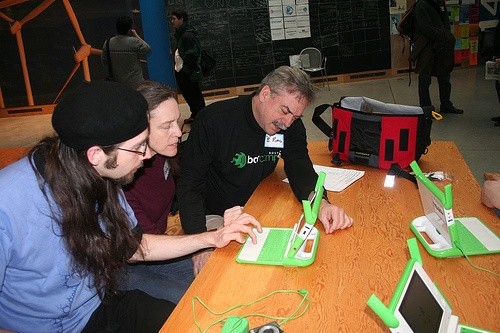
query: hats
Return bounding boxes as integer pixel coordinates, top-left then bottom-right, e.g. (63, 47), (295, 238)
(51, 81), (150, 145)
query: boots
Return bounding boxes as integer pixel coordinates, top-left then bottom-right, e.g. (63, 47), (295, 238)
(439, 85), (464, 115)
(417, 90), (441, 120)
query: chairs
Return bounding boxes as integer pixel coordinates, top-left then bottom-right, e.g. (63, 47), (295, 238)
(299, 48), (330, 91)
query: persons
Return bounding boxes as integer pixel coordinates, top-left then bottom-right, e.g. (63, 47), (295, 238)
(412, 0), (463, 118)
(490, 1), (500, 128)
(0, 83), (262, 333)
(104, 81), (194, 306)
(100, 15), (152, 90)
(168, 65), (354, 279)
(170, 9), (206, 124)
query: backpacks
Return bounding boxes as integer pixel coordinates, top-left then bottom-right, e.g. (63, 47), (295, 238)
(396, 0), (417, 35)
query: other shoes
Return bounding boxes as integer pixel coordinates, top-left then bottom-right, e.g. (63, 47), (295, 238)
(491, 116), (500, 126)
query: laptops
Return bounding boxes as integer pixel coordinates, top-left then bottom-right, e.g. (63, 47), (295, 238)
(409, 160), (500, 259)
(235, 171), (326, 267)
(367, 237), (497, 333)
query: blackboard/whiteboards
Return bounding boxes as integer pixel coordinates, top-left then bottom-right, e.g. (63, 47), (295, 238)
(165, 0), (392, 100)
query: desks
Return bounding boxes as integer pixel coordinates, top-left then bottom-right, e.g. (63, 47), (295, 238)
(158, 141), (500, 333)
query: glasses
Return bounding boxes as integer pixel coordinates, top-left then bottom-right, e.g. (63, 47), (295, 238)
(99, 122), (151, 157)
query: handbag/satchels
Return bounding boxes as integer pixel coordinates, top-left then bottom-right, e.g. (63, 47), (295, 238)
(312, 95), (443, 187)
(199, 50), (216, 76)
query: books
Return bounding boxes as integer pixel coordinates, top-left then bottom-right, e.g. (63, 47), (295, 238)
(484, 61), (499, 80)
